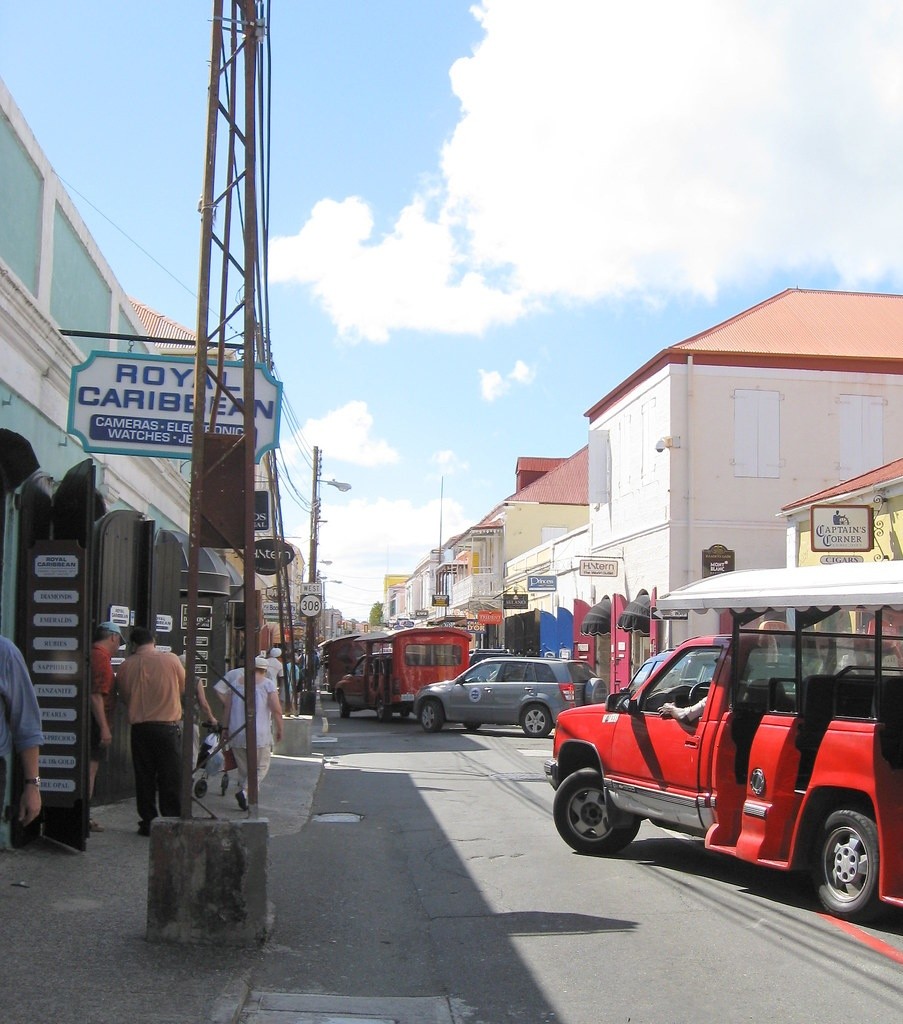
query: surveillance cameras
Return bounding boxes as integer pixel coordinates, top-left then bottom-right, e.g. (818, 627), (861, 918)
(656, 437), (672, 452)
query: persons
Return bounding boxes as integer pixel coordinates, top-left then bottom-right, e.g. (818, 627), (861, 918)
(0, 635), (46, 827)
(174, 654), (218, 770)
(212, 667), (244, 705)
(116, 627), (186, 836)
(221, 656), (283, 811)
(658, 653), (719, 723)
(265, 648), (320, 716)
(90, 622), (127, 832)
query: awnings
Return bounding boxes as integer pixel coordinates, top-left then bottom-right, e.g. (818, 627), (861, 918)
(580, 599), (612, 636)
(616, 593), (650, 635)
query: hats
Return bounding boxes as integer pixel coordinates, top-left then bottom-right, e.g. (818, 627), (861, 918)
(294, 656), (300, 664)
(269, 647), (282, 657)
(97, 622), (126, 646)
(254, 658), (270, 670)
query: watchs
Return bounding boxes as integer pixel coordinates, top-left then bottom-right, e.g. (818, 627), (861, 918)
(22, 776), (41, 786)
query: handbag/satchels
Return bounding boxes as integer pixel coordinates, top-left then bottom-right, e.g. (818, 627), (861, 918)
(197, 733), (225, 774)
(296, 680), (304, 692)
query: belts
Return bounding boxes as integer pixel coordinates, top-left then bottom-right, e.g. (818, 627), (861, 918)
(145, 721), (176, 726)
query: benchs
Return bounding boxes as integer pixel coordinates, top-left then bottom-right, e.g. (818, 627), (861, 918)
(729, 675), (903, 772)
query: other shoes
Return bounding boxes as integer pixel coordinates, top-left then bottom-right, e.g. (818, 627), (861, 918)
(138, 824), (151, 836)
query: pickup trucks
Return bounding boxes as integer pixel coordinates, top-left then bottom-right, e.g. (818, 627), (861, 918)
(543, 561), (903, 925)
(318, 625), (473, 723)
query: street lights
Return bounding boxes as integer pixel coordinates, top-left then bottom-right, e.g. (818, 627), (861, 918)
(299, 446), (352, 715)
(302, 560), (333, 583)
(323, 581), (343, 641)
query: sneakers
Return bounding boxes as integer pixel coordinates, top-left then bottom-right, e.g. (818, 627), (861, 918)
(235, 790), (248, 811)
(88, 819), (105, 832)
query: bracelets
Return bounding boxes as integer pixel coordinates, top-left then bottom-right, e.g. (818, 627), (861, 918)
(222, 727), (228, 730)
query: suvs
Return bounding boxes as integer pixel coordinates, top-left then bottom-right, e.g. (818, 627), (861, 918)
(413, 655), (608, 733)
(619, 646), (721, 699)
(468, 647), (513, 667)
